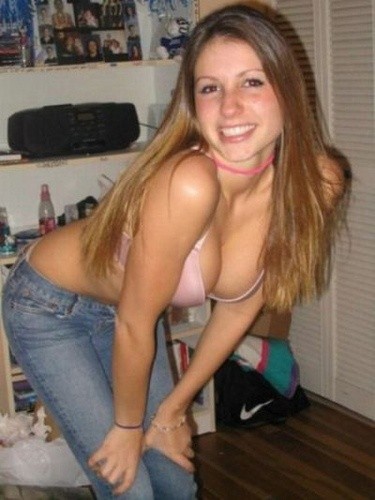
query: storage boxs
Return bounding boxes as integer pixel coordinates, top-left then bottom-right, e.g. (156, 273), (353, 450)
(248, 307), (292, 340)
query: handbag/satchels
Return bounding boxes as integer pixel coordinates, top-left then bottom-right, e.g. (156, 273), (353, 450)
(7, 102), (140, 159)
(171, 322), (301, 434)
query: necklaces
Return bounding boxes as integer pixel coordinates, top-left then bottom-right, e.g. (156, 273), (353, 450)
(206, 151), (274, 176)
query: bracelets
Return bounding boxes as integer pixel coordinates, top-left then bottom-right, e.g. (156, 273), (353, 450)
(113, 417), (145, 429)
(151, 414), (187, 432)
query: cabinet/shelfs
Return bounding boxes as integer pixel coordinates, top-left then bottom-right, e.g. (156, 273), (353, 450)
(0, 0), (217, 438)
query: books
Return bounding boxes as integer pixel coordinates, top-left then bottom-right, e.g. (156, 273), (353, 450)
(166, 339), (207, 406)
(10, 360), (37, 400)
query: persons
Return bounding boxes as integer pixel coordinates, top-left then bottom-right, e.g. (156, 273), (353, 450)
(0, 4), (352, 500)
(35, 1), (142, 65)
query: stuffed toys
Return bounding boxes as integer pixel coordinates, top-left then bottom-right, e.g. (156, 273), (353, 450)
(157, 17), (192, 62)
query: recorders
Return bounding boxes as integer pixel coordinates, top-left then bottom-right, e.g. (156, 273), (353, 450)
(7, 102), (141, 158)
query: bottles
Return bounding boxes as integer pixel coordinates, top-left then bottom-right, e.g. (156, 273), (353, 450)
(0, 208), (10, 247)
(39, 185), (55, 235)
(20, 33), (30, 68)
